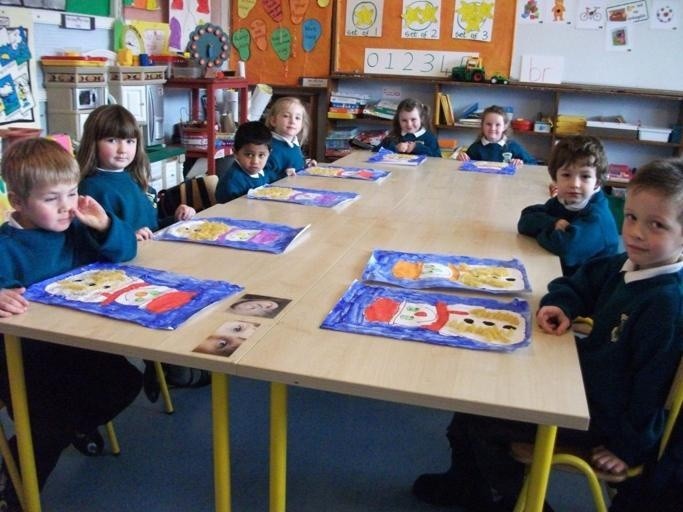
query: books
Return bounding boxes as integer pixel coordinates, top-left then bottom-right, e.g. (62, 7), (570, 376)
(325, 89), (398, 123)
(555, 114), (673, 147)
(325, 125), (388, 152)
(435, 92), (483, 128)
(606, 164), (632, 184)
(175, 119), (236, 146)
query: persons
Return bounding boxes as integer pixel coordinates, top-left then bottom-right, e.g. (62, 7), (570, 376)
(408, 156), (682, 511)
(76, 105), (195, 241)
(379, 95), (442, 157)
(515, 135), (619, 277)
(213, 120), (296, 203)
(0, 137), (146, 510)
(455, 104), (537, 165)
(263, 97), (318, 178)
(192, 319), (260, 358)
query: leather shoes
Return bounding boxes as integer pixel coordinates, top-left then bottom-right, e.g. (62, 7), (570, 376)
(71, 435), (104, 456)
(162, 364), (210, 386)
(412, 465), (467, 503)
(142, 363), (160, 401)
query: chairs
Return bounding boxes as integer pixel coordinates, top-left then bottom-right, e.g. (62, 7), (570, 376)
(511, 359), (682, 512)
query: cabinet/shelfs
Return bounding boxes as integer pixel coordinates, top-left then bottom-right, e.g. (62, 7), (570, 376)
(322, 72), (682, 193)
(107, 64), (170, 151)
(165, 78), (253, 176)
(42, 64), (108, 143)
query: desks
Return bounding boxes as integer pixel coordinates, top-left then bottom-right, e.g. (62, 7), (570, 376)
(0, 146), (591, 512)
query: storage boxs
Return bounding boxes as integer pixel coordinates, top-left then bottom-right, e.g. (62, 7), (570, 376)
(583, 119), (638, 142)
(638, 127), (672, 144)
